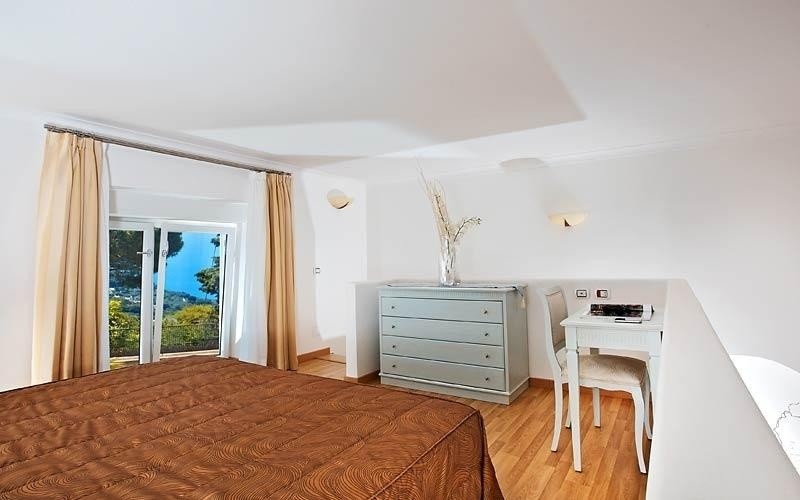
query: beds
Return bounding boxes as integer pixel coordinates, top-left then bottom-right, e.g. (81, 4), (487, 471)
(0, 356), (504, 500)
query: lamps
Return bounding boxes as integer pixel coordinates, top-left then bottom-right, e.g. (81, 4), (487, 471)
(550, 211), (584, 227)
(328, 189), (354, 210)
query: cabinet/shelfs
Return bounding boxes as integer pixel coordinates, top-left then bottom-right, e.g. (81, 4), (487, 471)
(377, 281), (529, 406)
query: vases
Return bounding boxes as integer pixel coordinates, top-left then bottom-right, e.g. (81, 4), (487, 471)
(440, 234), (456, 286)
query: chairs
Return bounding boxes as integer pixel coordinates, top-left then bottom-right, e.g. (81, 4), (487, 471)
(534, 285), (653, 473)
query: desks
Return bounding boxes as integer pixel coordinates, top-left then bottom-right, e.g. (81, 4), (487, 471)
(559, 303), (665, 474)
